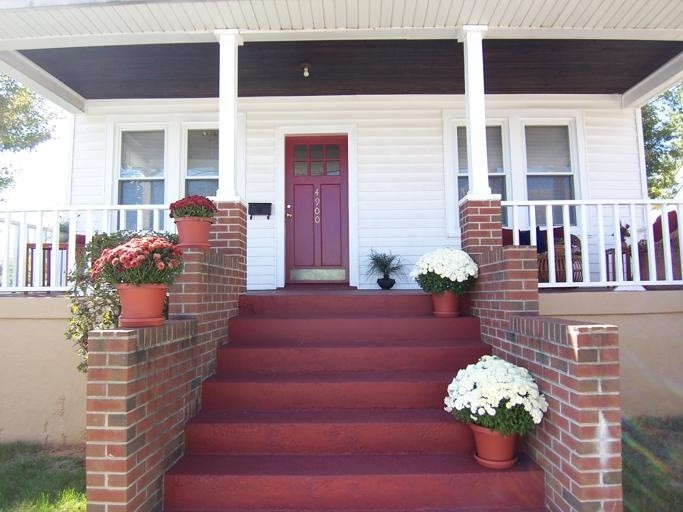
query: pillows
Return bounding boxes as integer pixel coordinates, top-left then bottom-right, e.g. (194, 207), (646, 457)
(502, 228), (520, 247)
(541, 227), (564, 251)
(519, 225), (543, 253)
(652, 210), (678, 243)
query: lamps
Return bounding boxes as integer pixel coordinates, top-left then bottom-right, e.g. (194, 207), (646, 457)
(301, 62), (312, 80)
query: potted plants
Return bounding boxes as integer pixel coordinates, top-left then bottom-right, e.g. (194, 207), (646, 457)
(611, 222), (631, 249)
(364, 250), (413, 292)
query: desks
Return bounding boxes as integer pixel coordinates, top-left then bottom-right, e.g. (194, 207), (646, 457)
(25, 242), (85, 294)
(606, 248), (632, 281)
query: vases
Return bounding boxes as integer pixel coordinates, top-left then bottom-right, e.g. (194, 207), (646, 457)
(468, 421), (518, 469)
(431, 290), (463, 319)
(116, 283), (168, 327)
(173, 217), (214, 250)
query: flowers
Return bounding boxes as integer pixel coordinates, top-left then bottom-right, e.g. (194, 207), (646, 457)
(170, 194), (218, 220)
(89, 236), (185, 288)
(443, 354), (550, 440)
(412, 247), (479, 297)
